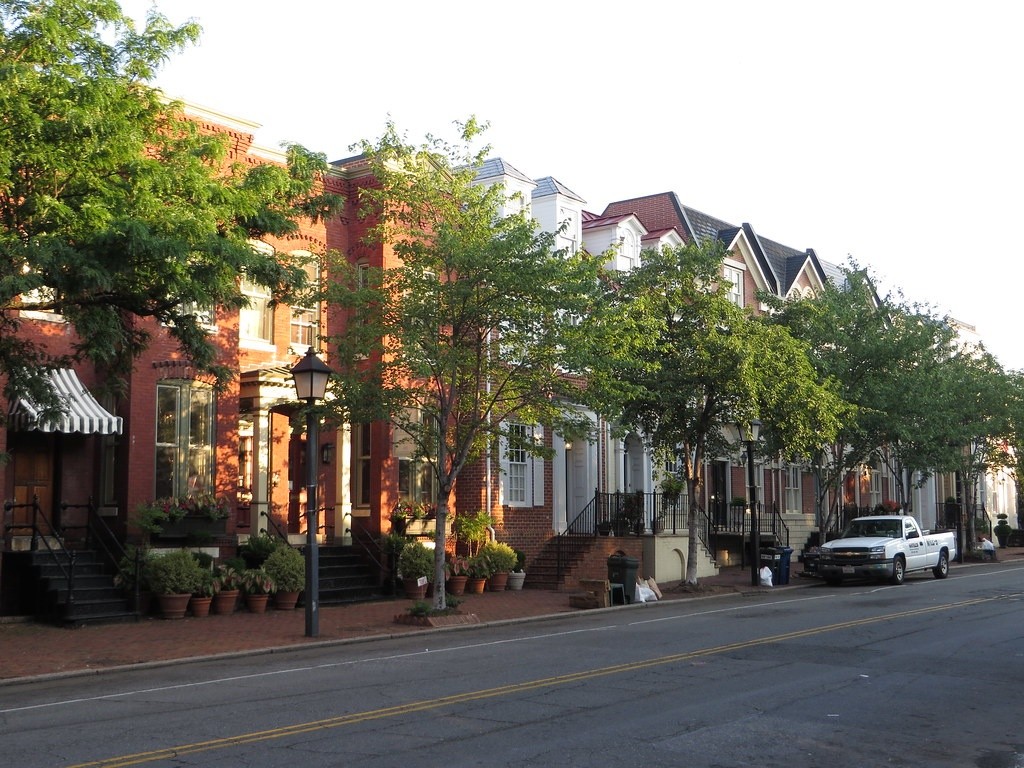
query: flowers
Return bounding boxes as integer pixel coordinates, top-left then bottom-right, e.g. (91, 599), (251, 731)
(610, 489), (645, 538)
(388, 497), (454, 524)
(151, 493), (231, 523)
(875, 499), (902, 513)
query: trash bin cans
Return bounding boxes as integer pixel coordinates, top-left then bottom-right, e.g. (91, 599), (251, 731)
(759, 547), (781, 585)
(775, 546), (794, 585)
(607, 555), (639, 605)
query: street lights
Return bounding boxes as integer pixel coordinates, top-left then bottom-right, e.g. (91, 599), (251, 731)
(290, 345), (335, 638)
(736, 416), (763, 587)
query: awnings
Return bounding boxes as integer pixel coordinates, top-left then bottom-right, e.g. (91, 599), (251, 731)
(8, 368), (123, 435)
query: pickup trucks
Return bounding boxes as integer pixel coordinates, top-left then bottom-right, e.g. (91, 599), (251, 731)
(814, 514), (957, 586)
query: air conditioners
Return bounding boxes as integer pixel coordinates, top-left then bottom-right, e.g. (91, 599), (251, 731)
(943, 497), (960, 530)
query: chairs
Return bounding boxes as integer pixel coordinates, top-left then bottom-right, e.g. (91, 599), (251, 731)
(866, 524), (877, 534)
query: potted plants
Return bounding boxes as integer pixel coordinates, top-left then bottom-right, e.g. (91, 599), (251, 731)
(373, 532), (417, 597)
(649, 470), (686, 534)
(263, 542), (306, 610)
(189, 568), (221, 618)
(221, 554), (247, 609)
(445, 559), (477, 597)
(597, 522), (612, 537)
(507, 550), (527, 591)
(993, 520), (1013, 546)
(240, 564), (278, 615)
(396, 541), (435, 599)
(730, 495), (749, 524)
(478, 540), (519, 591)
(632, 496), (647, 534)
(119, 543), (209, 619)
(463, 553), (498, 594)
(210, 564), (246, 615)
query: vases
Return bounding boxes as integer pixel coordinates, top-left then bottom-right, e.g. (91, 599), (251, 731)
(151, 516), (230, 538)
(397, 515), (452, 535)
(884, 507), (902, 512)
(611, 520), (629, 537)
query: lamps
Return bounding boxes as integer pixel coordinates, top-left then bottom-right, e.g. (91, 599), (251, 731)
(564, 439), (574, 450)
